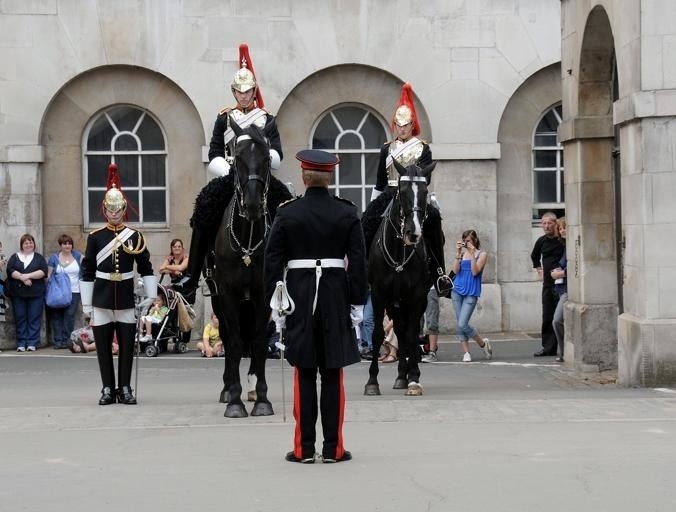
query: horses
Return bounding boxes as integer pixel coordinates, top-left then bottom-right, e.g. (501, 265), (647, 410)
(203, 115), (276, 417)
(364, 155), (438, 396)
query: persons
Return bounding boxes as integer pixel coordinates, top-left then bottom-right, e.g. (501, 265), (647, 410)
(550, 215), (566, 361)
(196, 284), (440, 363)
(530, 212), (564, 358)
(78, 187), (158, 404)
(171, 68), (293, 306)
(134, 292), (169, 342)
(450, 229), (494, 363)
(0, 241), (8, 323)
(66, 329), (119, 355)
(5, 233), (49, 352)
(262, 148), (371, 464)
(359, 105), (454, 299)
(43, 234), (85, 350)
(158, 238), (196, 353)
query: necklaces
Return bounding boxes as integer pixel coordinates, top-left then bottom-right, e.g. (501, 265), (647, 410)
(65, 260), (69, 264)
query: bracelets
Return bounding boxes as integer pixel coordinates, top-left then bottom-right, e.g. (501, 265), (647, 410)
(455, 255), (461, 260)
(171, 270), (175, 275)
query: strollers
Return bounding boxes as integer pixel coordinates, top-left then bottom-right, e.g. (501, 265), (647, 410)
(129, 269), (189, 357)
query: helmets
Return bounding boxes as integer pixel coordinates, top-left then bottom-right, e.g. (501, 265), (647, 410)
(392, 83), (420, 137)
(101, 164), (128, 226)
(231, 44), (264, 108)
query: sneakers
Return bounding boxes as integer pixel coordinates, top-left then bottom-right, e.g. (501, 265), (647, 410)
(360, 350), (397, 363)
(201, 349), (207, 357)
(463, 351), (472, 362)
(27, 346), (36, 351)
(17, 347), (25, 352)
(420, 351), (438, 363)
(217, 351), (224, 357)
(140, 334), (151, 342)
(479, 337), (492, 360)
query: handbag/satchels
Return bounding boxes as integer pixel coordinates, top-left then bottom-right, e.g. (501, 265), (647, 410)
(175, 292), (197, 333)
(44, 253), (72, 309)
(445, 255), (463, 298)
(4, 253), (17, 297)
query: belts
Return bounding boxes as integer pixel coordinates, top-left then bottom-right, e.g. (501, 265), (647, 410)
(287, 259), (346, 269)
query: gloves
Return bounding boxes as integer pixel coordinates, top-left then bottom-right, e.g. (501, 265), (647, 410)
(272, 309), (286, 333)
(83, 306), (94, 320)
(137, 297), (155, 316)
(350, 303), (365, 330)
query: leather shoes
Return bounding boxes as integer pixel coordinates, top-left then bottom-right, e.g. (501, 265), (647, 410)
(436, 280), (450, 297)
(534, 349), (557, 356)
(285, 451), (315, 463)
(322, 450), (352, 463)
(99, 386), (116, 405)
(172, 276), (196, 291)
(115, 385), (136, 404)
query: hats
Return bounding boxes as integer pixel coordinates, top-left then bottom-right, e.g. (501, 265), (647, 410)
(295, 150), (341, 172)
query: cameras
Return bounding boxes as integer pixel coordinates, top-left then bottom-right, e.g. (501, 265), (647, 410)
(460, 242), (467, 247)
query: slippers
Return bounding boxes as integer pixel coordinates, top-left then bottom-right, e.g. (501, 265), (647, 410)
(78, 338), (85, 353)
(66, 338), (75, 353)
